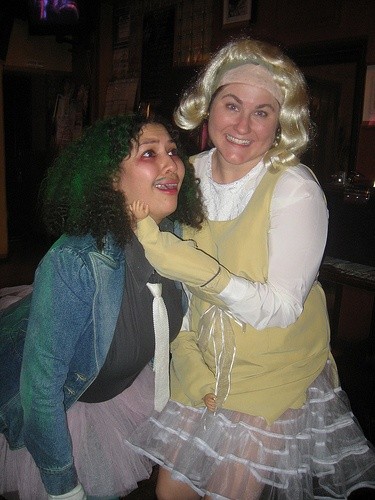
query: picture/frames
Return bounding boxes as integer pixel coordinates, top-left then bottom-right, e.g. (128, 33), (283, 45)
(218, 0), (256, 31)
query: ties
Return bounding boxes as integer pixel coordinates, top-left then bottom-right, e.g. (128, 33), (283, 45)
(146, 282), (169, 413)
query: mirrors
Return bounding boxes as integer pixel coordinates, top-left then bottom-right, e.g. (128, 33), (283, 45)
(285, 33), (367, 186)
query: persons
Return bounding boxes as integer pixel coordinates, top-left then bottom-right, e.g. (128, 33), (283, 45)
(155, 39), (375, 500)
(0, 114), (208, 500)
